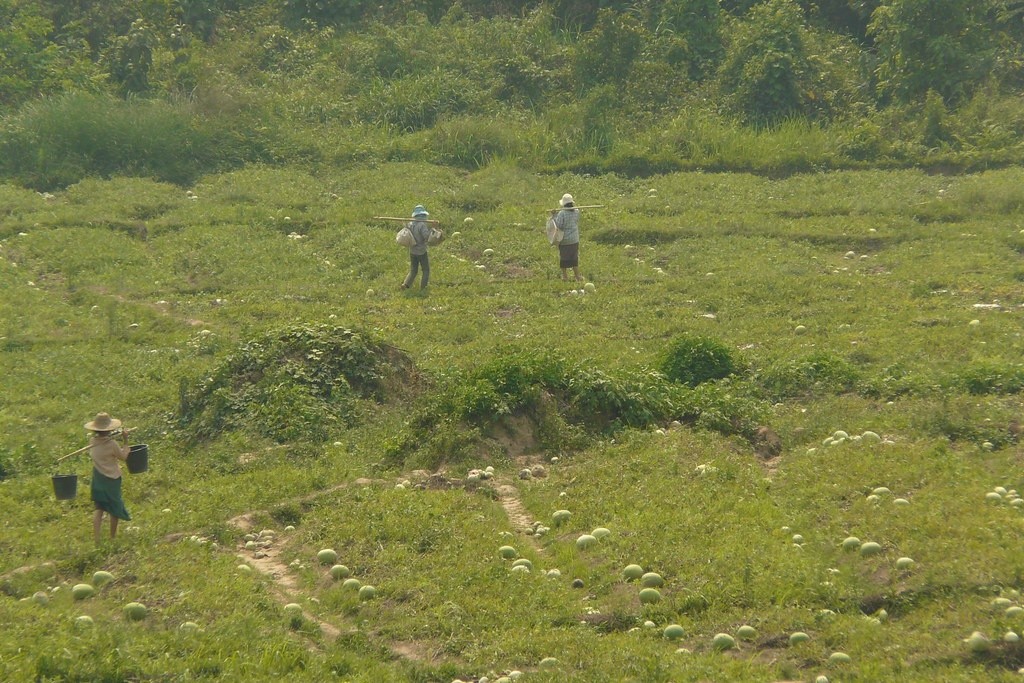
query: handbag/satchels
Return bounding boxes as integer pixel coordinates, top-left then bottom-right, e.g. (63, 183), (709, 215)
(427, 228), (446, 247)
(395, 222), (418, 249)
(546, 218), (565, 246)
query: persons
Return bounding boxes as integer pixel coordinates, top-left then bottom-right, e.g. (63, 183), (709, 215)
(401, 205), (438, 291)
(89, 413), (130, 544)
(550, 193), (584, 282)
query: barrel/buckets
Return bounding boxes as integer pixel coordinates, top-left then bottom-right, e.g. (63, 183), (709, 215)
(125, 428), (149, 474)
(51, 455), (77, 500)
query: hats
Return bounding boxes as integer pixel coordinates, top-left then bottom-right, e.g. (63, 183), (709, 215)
(83, 412), (122, 431)
(558, 193), (576, 206)
(411, 204), (429, 217)
